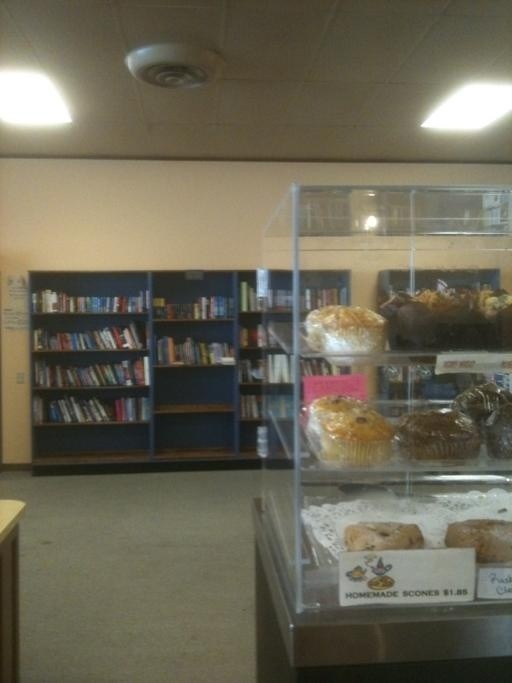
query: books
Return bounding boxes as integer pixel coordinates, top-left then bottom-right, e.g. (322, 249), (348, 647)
(152, 294), (233, 365)
(240, 280), (347, 420)
(32, 287), (150, 424)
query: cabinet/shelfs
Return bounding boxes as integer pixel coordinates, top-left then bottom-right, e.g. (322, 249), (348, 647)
(28, 268), (153, 474)
(150, 268), (237, 472)
(237, 186), (512, 668)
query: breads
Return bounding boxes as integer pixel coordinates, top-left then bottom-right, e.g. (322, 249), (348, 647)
(446, 519), (511, 563)
(346, 520), (426, 550)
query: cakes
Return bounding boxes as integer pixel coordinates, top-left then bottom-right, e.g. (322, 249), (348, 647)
(304, 279), (512, 353)
(394, 407), (481, 462)
(318, 406), (394, 461)
(452, 383), (512, 445)
(483, 402), (511, 460)
(310, 395), (366, 436)
